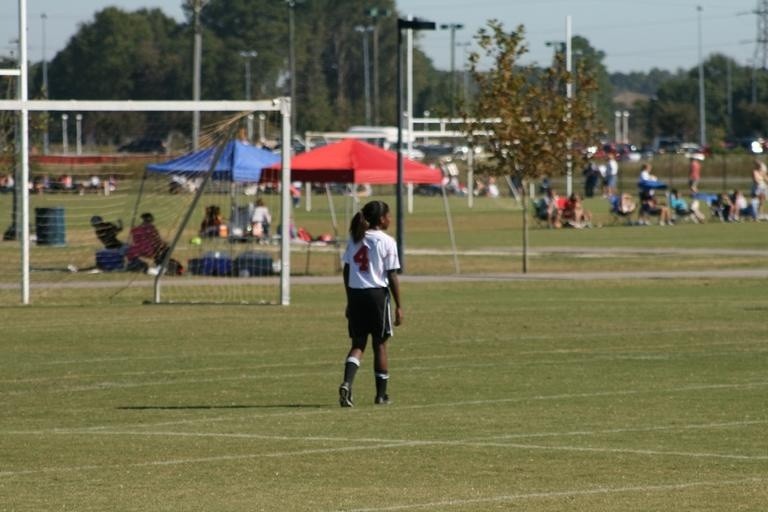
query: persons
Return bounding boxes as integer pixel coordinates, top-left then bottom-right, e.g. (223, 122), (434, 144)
(0, 174), (101, 197)
(169, 174), (187, 193)
(415, 135), (768, 230)
(446, 175), (550, 196)
(92, 212), (164, 268)
(338, 199), (405, 408)
(289, 185), (300, 208)
(584, 154), (617, 198)
(535, 187), (591, 230)
(201, 199), (272, 239)
(608, 157), (767, 227)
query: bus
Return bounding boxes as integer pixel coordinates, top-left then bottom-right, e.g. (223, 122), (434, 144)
(303, 126), (425, 160)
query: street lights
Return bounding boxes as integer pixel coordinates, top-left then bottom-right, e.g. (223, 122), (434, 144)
(439, 23), (462, 116)
(39, 13), (50, 188)
(352, 24), (374, 126)
(239, 50), (257, 100)
(695, 5), (706, 147)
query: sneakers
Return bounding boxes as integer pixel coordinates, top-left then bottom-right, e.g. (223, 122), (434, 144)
(374, 396), (394, 405)
(339, 382), (353, 407)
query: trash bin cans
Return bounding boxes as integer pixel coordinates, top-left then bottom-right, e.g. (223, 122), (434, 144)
(35, 207), (65, 245)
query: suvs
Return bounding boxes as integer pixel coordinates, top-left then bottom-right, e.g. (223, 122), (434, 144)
(583, 143), (642, 161)
